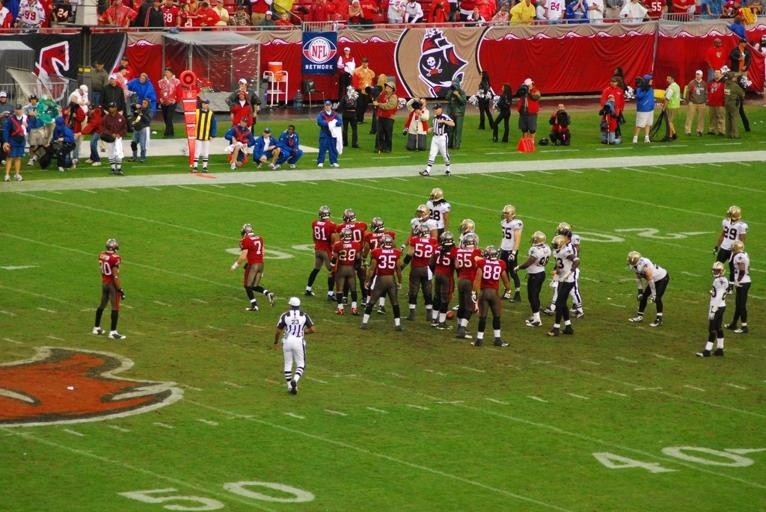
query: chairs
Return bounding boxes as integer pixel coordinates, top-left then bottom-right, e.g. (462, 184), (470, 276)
(301, 79), (326, 110)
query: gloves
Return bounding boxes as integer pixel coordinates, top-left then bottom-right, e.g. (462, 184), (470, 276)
(120, 288), (126, 299)
(713, 246), (718, 256)
(651, 296), (656, 301)
(637, 294), (643, 301)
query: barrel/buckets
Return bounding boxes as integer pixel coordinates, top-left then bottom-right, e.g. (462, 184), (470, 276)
(268, 61), (283, 81)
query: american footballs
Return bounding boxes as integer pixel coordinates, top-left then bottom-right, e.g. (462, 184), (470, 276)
(446, 310), (454, 318)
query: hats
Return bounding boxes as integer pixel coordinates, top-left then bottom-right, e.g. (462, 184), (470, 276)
(522, 78), (534, 86)
(202, 78), (333, 134)
(607, 38), (747, 103)
(432, 102), (441, 109)
(0, 56), (172, 130)
(384, 80), (396, 92)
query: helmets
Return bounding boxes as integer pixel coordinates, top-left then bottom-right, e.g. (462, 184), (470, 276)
(712, 261), (724, 277)
(628, 251), (640, 266)
(731, 241), (744, 253)
(106, 239), (119, 250)
(319, 187), (572, 260)
(727, 206), (742, 221)
(243, 224), (253, 233)
(288, 297), (300, 306)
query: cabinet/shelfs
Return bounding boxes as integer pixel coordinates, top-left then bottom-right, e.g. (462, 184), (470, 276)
(263, 70), (288, 107)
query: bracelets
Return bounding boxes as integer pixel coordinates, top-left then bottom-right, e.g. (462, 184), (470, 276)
(714, 246), (718, 251)
(118, 288), (124, 293)
(231, 262), (240, 270)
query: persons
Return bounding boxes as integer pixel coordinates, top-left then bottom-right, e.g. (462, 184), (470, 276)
(477, 77), (571, 146)
(317, 48), (466, 177)
(1, 1), (288, 33)
(598, 68), (626, 145)
(724, 240), (751, 333)
(192, 99), (217, 173)
(631, 38), (752, 143)
(274, 297), (315, 394)
(0, 57), (156, 182)
(92, 239), (126, 340)
(713, 206), (748, 295)
(497, 205), (523, 302)
(426, 188), (451, 240)
(229, 223), (275, 311)
(399, 204), (510, 346)
(514, 221), (583, 336)
(225, 79), (303, 171)
(305, 206), (401, 331)
(158, 69), (182, 137)
(696, 261), (729, 357)
(627, 251), (669, 327)
(348, 1), (766, 30)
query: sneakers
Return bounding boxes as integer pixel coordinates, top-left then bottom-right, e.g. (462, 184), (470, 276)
(268, 293), (275, 305)
(649, 320), (663, 327)
(108, 329), (126, 340)
(696, 282), (748, 357)
(93, 327), (105, 335)
(492, 132), (738, 145)
(629, 316), (643, 322)
(288, 380), (297, 394)
(446, 166), (450, 173)
(1, 154), (144, 181)
(306, 286), (584, 346)
(193, 162), (341, 173)
(418, 170), (429, 175)
(246, 305), (258, 311)
